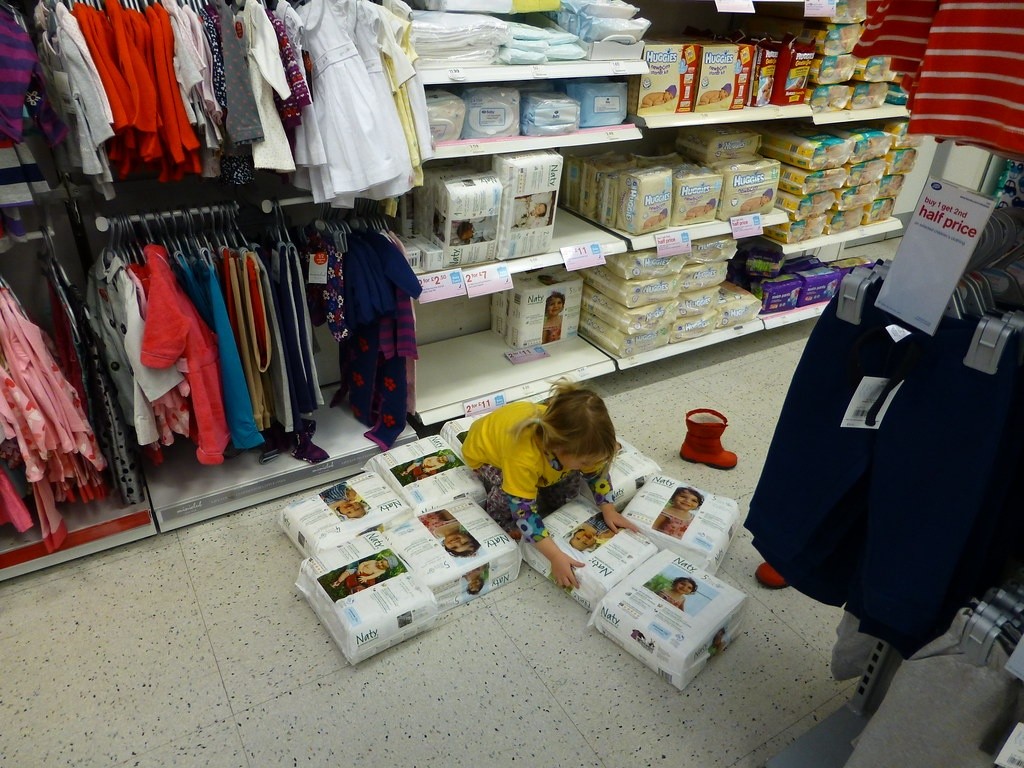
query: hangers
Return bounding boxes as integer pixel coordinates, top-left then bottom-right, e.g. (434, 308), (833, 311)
(36, 228), (90, 331)
(839, 190), (1024, 378)
(0, 273), (23, 313)
(305, 187), (391, 236)
(958, 582), (1023, 684)
(104, 198), (296, 287)
(46, 0), (316, 14)
(377, 0), (414, 19)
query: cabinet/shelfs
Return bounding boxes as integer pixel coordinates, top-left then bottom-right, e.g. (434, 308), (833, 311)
(35, 0), (421, 534)
(593, 0), (811, 373)
(361, 0), (643, 429)
(739, 0), (904, 335)
(0, 0), (160, 585)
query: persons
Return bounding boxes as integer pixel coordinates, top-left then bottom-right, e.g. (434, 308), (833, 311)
(652, 486), (705, 540)
(789, 76), (805, 88)
(736, 188), (773, 215)
(698, 83), (732, 106)
(417, 509), (480, 558)
(708, 627), (726, 661)
(569, 512), (609, 552)
(457, 215), (498, 243)
(512, 195), (547, 229)
(658, 576), (698, 612)
(643, 208), (668, 229)
(762, 76), (773, 99)
(319, 482), (366, 519)
(543, 291), (565, 344)
(684, 198), (716, 220)
(461, 378), (639, 589)
(640, 85), (677, 108)
(400, 454), (455, 481)
(463, 564), (488, 594)
(329, 555), (398, 594)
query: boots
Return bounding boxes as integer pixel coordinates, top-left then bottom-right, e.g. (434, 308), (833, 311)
(754, 561), (788, 588)
(680, 408), (738, 470)
